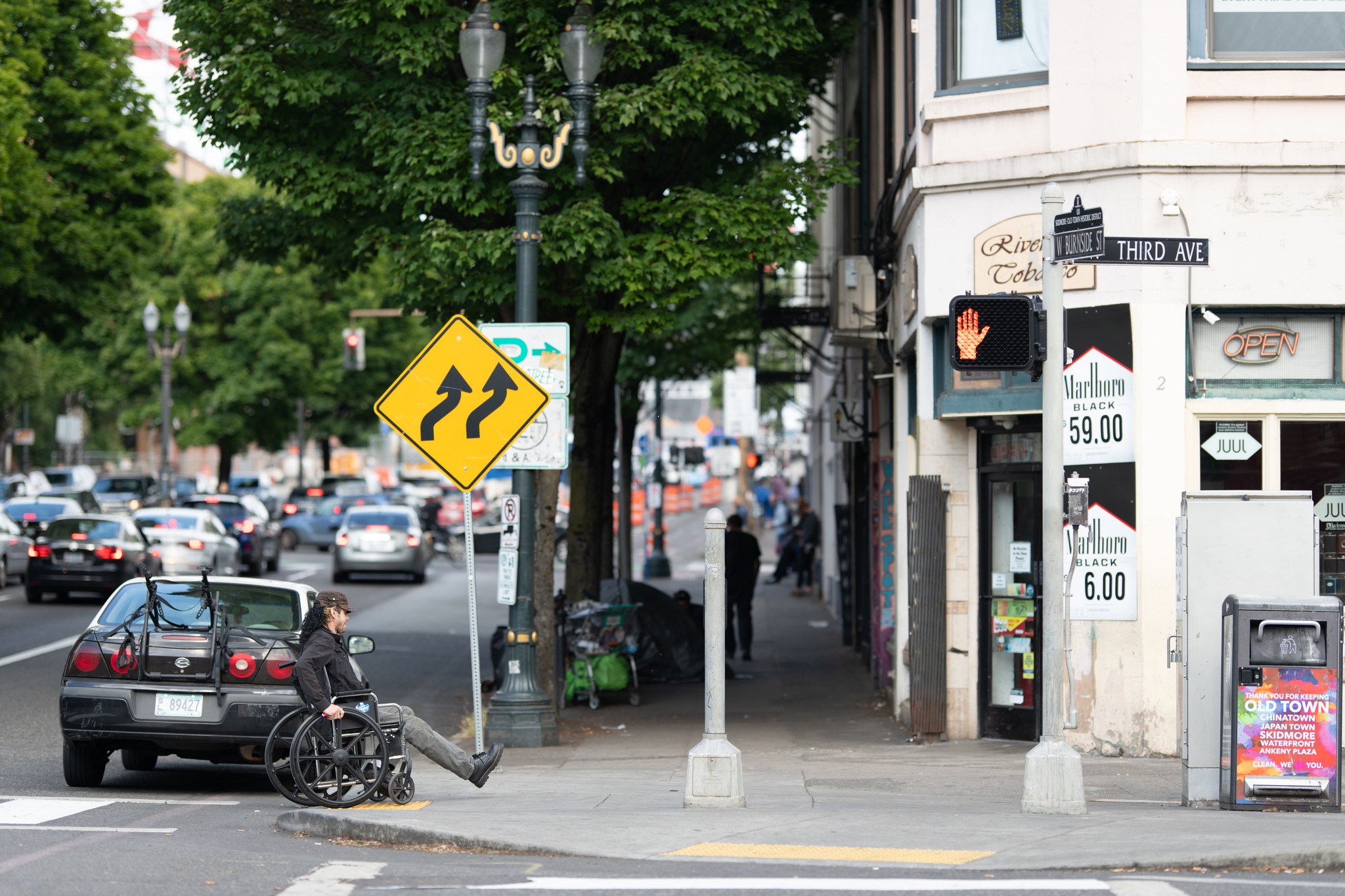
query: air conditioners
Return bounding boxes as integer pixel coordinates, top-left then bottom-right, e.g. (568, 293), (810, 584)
(829, 255), (878, 330)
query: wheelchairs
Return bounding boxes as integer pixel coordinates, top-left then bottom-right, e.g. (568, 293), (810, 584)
(264, 660), (415, 809)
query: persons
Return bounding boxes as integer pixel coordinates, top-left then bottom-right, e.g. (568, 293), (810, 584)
(675, 588), (703, 626)
(762, 499), (821, 596)
(422, 498), (449, 554)
(725, 514), (761, 662)
(290, 591), (504, 790)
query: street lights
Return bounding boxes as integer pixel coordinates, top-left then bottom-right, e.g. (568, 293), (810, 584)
(459, 1), (604, 747)
(142, 296), (189, 507)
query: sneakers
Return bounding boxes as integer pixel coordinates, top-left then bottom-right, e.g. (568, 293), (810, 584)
(363, 763), (394, 786)
(468, 743), (504, 788)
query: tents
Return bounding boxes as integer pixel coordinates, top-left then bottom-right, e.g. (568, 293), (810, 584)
(625, 577), (736, 682)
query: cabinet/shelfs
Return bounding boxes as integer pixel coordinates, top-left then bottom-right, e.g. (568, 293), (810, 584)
(1320, 531), (1345, 595)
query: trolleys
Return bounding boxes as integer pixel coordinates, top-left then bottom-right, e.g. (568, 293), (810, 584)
(558, 602), (643, 709)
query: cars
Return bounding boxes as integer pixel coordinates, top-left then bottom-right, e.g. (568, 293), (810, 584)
(0, 463), (569, 602)
(60, 562), (376, 787)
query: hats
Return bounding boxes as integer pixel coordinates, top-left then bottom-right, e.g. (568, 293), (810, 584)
(313, 590), (356, 614)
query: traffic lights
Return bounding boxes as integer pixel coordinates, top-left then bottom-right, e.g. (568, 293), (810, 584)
(951, 295), (1034, 371)
(344, 330), (364, 371)
